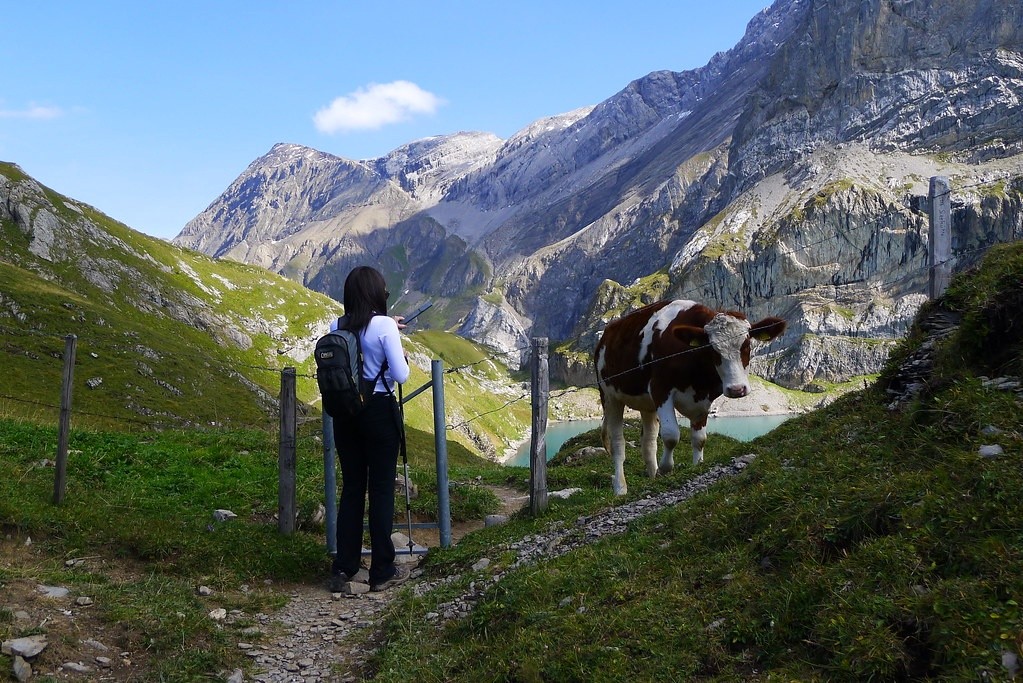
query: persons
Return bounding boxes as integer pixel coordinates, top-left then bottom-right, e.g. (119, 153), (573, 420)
(327, 267), (412, 590)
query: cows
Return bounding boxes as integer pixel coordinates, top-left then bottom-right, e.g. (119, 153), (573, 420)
(594, 300), (788, 496)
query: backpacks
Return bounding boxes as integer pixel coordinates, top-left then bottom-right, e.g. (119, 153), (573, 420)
(314, 312), (388, 418)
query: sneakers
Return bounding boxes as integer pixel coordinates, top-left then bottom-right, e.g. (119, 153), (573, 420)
(329, 569), (370, 592)
(370, 566), (409, 590)
